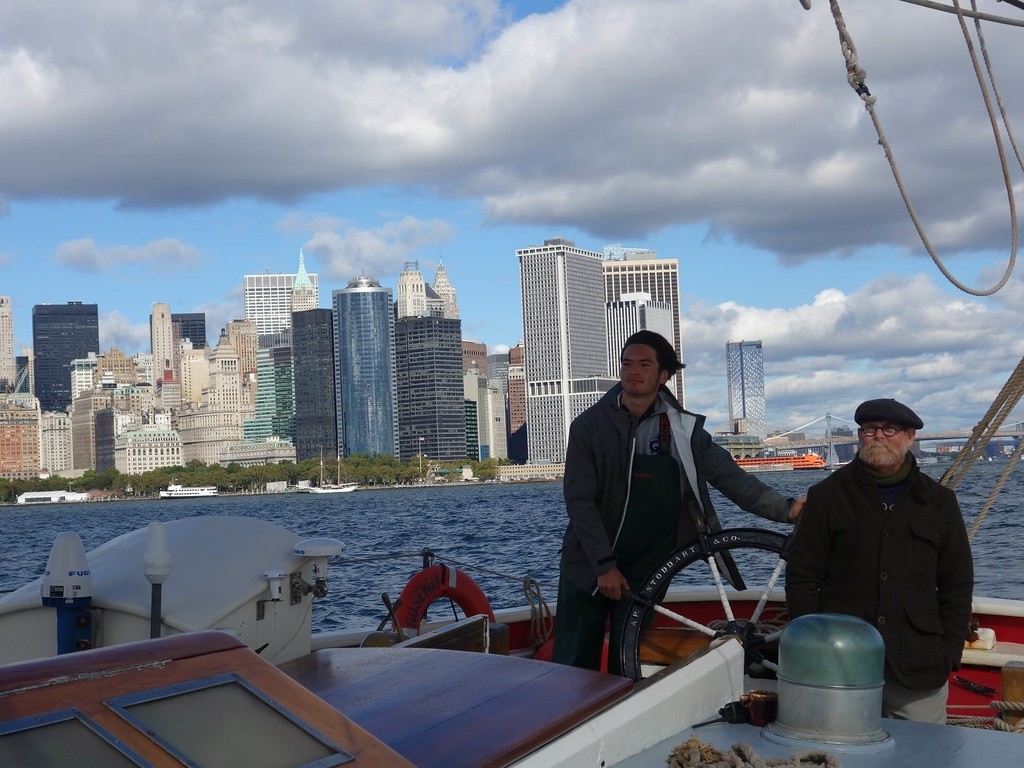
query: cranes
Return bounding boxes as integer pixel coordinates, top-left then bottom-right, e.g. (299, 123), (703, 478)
(28, 349), (35, 398)
(14, 352), (36, 393)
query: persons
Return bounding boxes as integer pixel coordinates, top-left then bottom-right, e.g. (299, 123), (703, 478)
(784, 398), (974, 724)
(551, 330), (807, 676)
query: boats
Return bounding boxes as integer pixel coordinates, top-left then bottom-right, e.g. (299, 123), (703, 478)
(17, 490), (90, 506)
(734, 449), (827, 473)
(159, 477), (219, 499)
(306, 446), (364, 494)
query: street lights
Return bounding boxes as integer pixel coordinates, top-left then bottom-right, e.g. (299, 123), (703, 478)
(419, 435), (424, 474)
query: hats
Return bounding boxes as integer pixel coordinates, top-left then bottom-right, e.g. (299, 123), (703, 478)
(855, 399), (923, 430)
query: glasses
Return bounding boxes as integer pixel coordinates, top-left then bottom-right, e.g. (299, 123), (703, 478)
(860, 425), (906, 437)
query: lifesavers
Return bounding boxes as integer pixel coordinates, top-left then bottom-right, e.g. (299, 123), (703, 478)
(388, 563), (498, 640)
